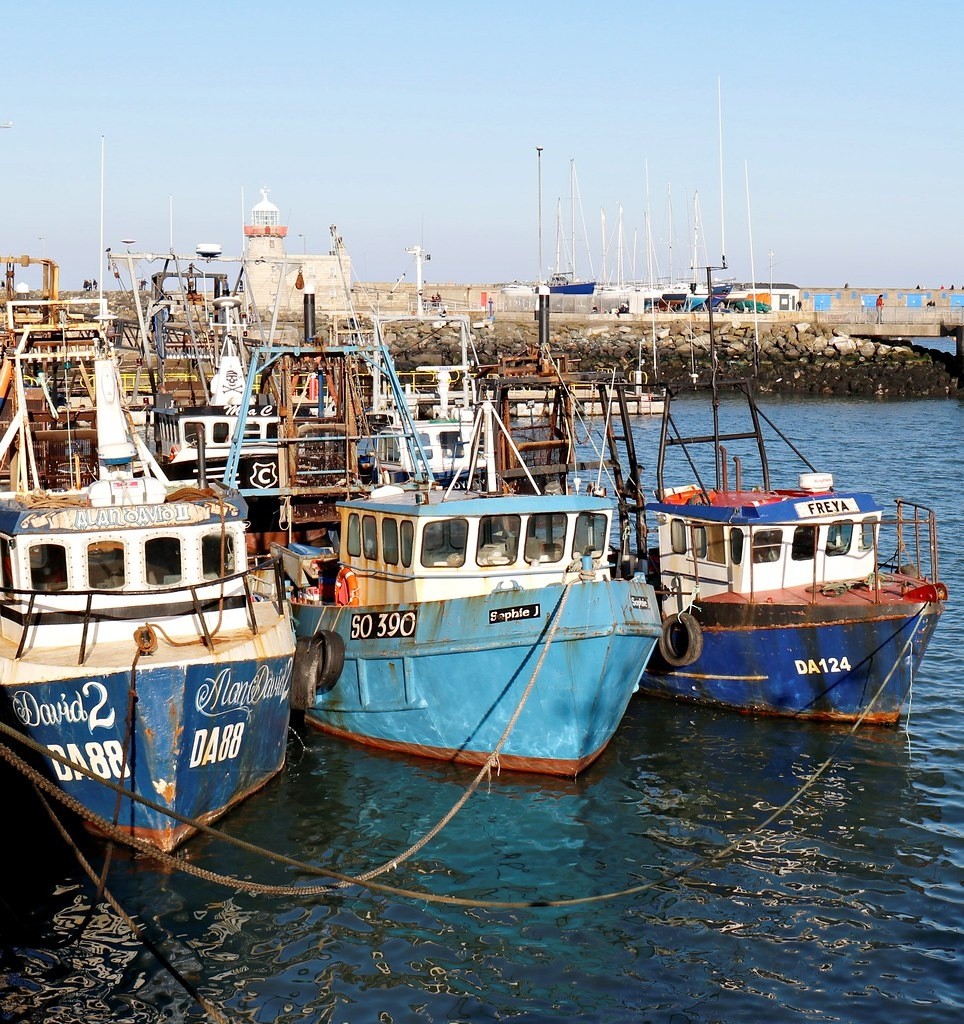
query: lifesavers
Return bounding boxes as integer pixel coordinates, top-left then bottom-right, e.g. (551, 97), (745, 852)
(332, 568), (360, 606)
(169, 446), (178, 462)
(748, 497), (793, 507)
(663, 489), (716, 506)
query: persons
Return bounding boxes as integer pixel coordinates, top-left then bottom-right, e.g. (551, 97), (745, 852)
(951, 285), (954, 289)
(876, 295), (884, 323)
(962, 286), (964, 289)
(617, 305), (629, 316)
(917, 285), (920, 289)
(240, 280), (243, 291)
(927, 300), (936, 306)
(139, 279), (148, 289)
(844, 283), (848, 288)
(84, 279), (98, 291)
(0, 281), (5, 288)
(797, 300), (802, 310)
(940, 284), (945, 290)
(224, 279), (229, 290)
(423, 292), (441, 307)
(593, 305), (597, 311)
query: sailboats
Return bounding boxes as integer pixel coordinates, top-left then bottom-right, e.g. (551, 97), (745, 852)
(516, 76), (946, 727)
(0, 134), (297, 852)
(170, 245), (665, 776)
(500, 157), (719, 297)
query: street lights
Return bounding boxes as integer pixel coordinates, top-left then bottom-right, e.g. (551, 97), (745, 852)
(535, 146), (544, 285)
